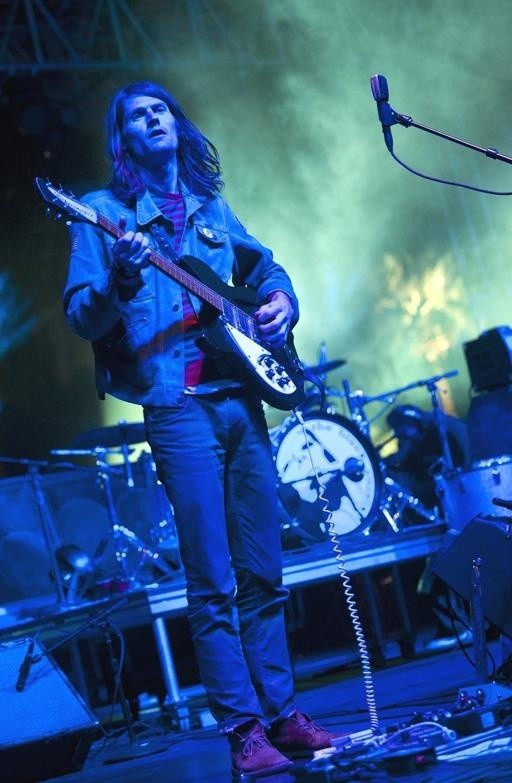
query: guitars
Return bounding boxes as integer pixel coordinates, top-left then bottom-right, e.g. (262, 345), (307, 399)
(32, 175), (304, 410)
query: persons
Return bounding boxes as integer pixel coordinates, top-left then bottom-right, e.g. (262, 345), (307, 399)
(388, 405), (471, 464)
(63, 82), (343, 779)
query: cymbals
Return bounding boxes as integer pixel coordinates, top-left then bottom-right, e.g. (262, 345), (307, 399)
(69, 422), (146, 449)
(307, 357), (346, 375)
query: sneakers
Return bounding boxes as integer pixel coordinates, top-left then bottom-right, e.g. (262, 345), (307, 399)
(228, 710), (350, 782)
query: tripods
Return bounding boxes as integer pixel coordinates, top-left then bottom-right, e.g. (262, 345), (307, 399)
(49, 445), (179, 607)
(341, 381), (442, 537)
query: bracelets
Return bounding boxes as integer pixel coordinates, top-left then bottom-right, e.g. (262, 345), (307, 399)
(118, 273), (141, 289)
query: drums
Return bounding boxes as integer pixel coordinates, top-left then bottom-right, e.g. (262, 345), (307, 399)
(270, 410), (385, 540)
(432, 456), (511, 532)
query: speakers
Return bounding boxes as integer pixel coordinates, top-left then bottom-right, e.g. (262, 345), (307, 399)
(0, 635), (99, 783)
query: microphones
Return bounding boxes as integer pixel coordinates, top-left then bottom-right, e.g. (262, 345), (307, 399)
(16, 642), (34, 688)
(370, 75), (393, 150)
